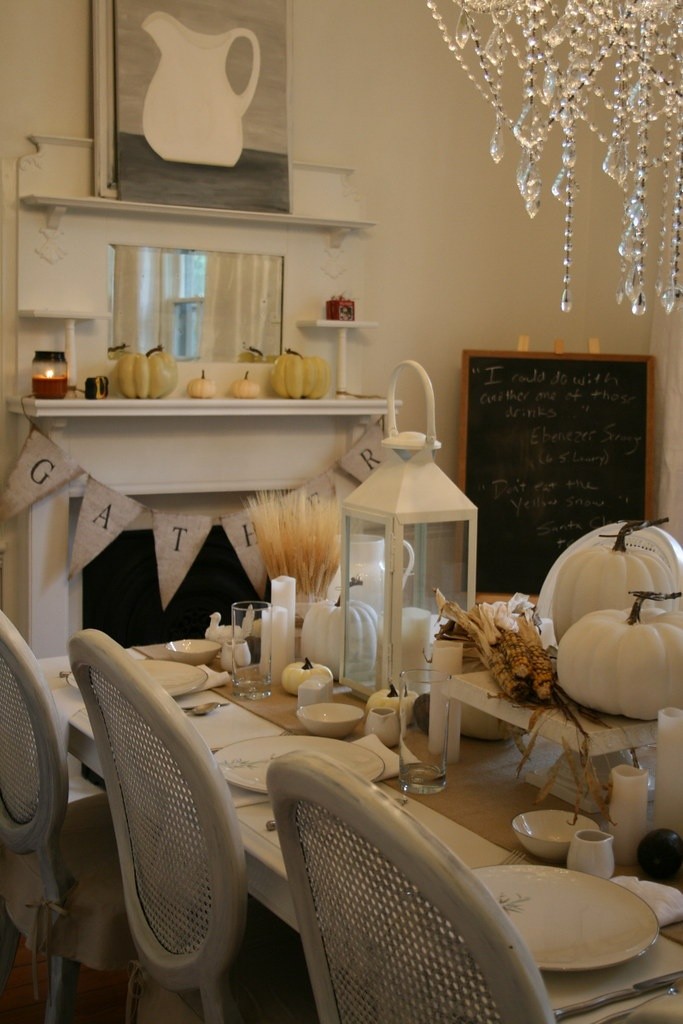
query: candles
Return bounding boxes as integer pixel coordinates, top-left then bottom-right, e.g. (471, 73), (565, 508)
(29, 351), (68, 399)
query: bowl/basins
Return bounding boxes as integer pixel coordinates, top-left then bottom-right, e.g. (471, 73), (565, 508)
(512, 809), (599, 860)
(297, 702), (365, 737)
(165, 639), (224, 667)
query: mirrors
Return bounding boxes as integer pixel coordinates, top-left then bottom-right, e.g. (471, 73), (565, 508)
(103, 242), (285, 367)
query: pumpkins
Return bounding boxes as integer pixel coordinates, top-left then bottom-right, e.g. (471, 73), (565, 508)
(232, 369), (261, 398)
(555, 590), (683, 721)
(270, 347), (334, 399)
(186, 370), (216, 397)
(365, 680), (419, 726)
(552, 511), (681, 644)
(281, 656), (336, 698)
(115, 347), (180, 400)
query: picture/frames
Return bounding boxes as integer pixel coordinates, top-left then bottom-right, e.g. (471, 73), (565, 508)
(89, 1), (295, 214)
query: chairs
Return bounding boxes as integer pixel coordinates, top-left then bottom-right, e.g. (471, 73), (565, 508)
(62, 626), (320, 1024)
(259, 749), (560, 1024)
(0, 612), (156, 1024)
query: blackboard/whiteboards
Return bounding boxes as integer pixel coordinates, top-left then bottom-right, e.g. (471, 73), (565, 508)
(454, 350), (658, 607)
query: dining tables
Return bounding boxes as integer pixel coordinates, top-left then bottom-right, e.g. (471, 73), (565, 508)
(20, 618), (682, 1024)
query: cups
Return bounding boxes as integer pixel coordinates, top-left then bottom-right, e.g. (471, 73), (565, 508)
(366, 706), (399, 746)
(568, 828), (618, 879)
(336, 532), (415, 614)
(31, 351), (68, 399)
(221, 639), (251, 671)
(297, 679), (327, 709)
(399, 668), (452, 795)
(232, 601), (273, 702)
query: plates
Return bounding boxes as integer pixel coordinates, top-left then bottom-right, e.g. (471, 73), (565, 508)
(69, 659), (209, 697)
(213, 735), (386, 794)
(468, 863), (659, 972)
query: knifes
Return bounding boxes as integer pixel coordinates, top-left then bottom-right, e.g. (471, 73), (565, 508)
(182, 702), (229, 712)
(549, 969), (656, 1022)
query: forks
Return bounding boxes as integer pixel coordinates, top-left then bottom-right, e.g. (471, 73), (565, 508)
(499, 849), (527, 866)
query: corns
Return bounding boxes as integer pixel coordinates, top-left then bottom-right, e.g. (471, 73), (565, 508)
(487, 630), (556, 701)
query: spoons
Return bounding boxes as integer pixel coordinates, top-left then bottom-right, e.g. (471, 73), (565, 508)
(185, 701), (220, 716)
(592, 978), (683, 1024)
